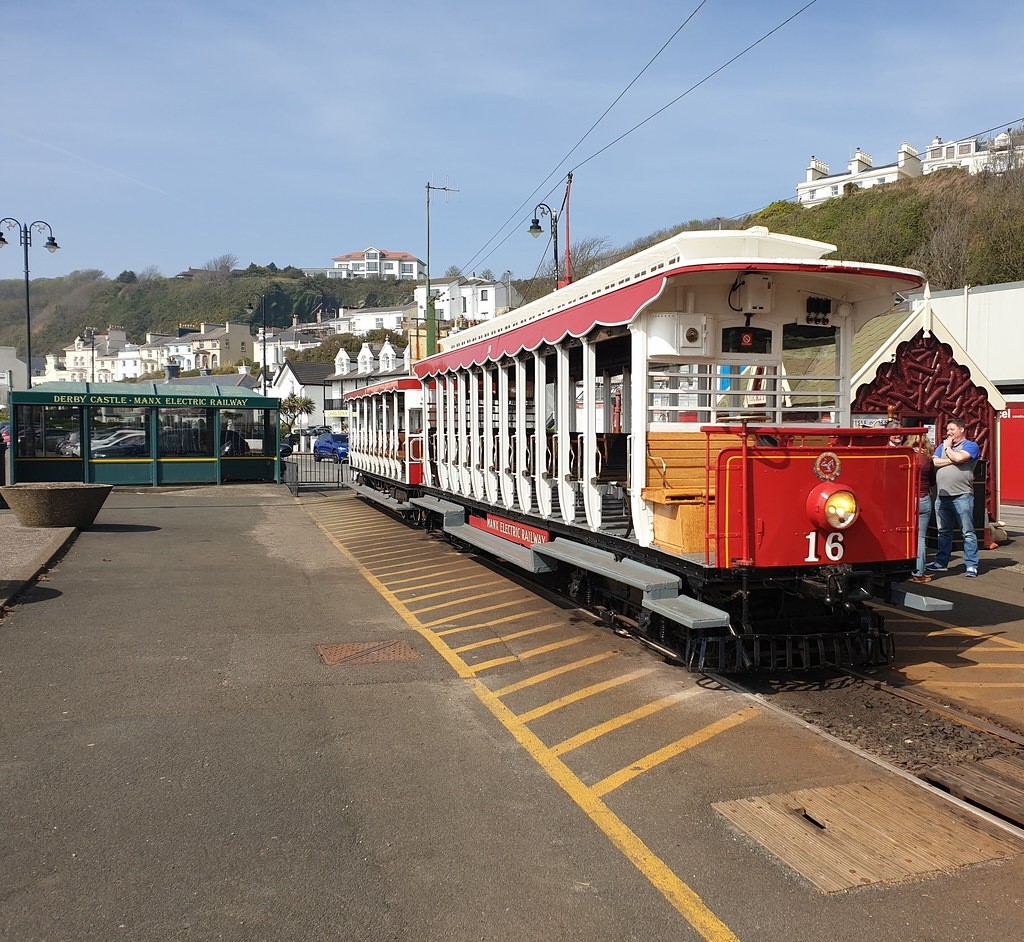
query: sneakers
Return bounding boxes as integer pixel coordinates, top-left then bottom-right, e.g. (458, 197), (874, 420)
(925, 562), (949, 572)
(965, 565), (977, 577)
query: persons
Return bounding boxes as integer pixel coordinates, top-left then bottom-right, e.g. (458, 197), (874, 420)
(886, 422), (904, 447)
(928, 420), (980, 577)
(905, 435), (934, 583)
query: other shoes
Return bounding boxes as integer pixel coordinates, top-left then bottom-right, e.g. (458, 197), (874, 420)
(913, 576), (931, 583)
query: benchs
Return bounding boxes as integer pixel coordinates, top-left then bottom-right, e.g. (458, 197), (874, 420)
(640, 431), (829, 557)
(351, 427), (632, 539)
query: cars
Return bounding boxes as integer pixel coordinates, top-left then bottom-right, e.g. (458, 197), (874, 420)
(280, 434), (311, 447)
(291, 428), (309, 437)
(307, 428), (333, 436)
(313, 433), (349, 463)
(0, 422), (146, 455)
(96, 428), (253, 459)
(243, 434), (293, 457)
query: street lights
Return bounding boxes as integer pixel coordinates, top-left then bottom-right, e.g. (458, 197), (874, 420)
(527, 203), (559, 292)
(0, 217), (62, 390)
(245, 293), (267, 398)
(79, 328), (94, 382)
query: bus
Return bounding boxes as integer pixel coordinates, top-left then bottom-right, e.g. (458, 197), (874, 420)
(343, 225), (933, 674)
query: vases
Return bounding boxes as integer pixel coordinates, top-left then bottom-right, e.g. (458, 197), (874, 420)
(0, 482), (114, 527)
